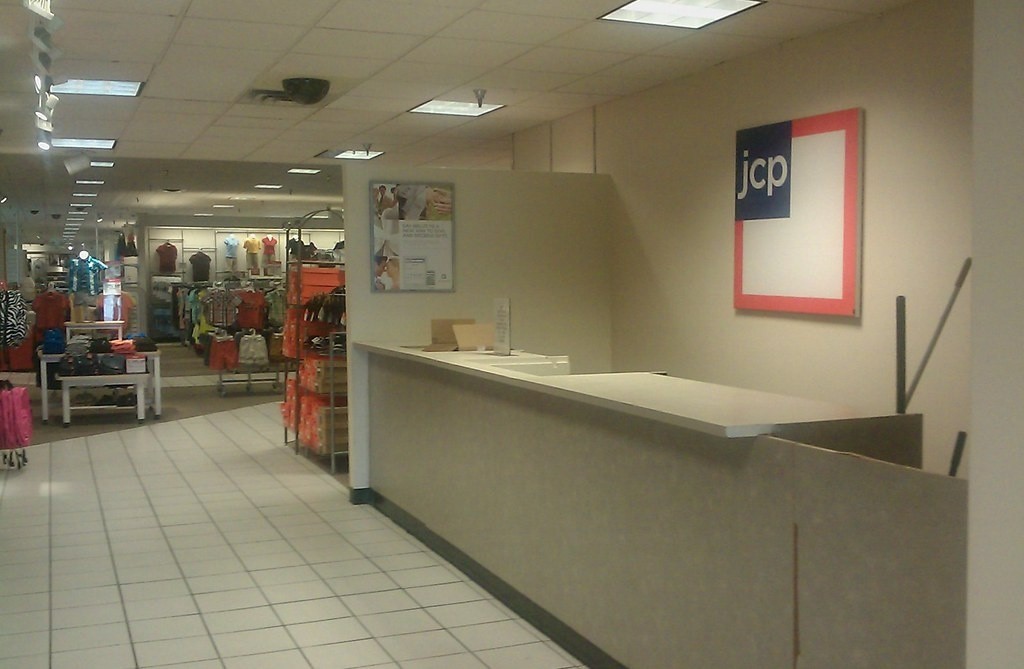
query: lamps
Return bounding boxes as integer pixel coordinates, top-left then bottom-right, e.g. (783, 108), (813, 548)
(31, 72), (58, 152)
(96, 212), (104, 222)
(62, 150), (89, 177)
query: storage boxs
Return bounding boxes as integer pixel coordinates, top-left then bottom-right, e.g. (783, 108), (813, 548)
(421, 317), (493, 353)
(279, 265), (346, 454)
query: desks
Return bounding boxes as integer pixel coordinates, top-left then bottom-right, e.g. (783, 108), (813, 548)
(35, 342), (162, 423)
(56, 373), (155, 424)
(63, 320), (124, 342)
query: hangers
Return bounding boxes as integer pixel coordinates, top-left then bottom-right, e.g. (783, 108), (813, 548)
(177, 275), (282, 296)
(210, 327), (284, 341)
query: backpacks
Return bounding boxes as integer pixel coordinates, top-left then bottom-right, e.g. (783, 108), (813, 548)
(0, 385), (33, 450)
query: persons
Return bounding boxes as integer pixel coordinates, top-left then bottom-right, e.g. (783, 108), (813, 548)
(0, 231), (138, 386)
(156, 233), (305, 282)
(374, 182), (452, 289)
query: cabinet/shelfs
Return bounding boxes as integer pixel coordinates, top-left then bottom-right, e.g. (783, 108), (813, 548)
(281, 260), (347, 474)
